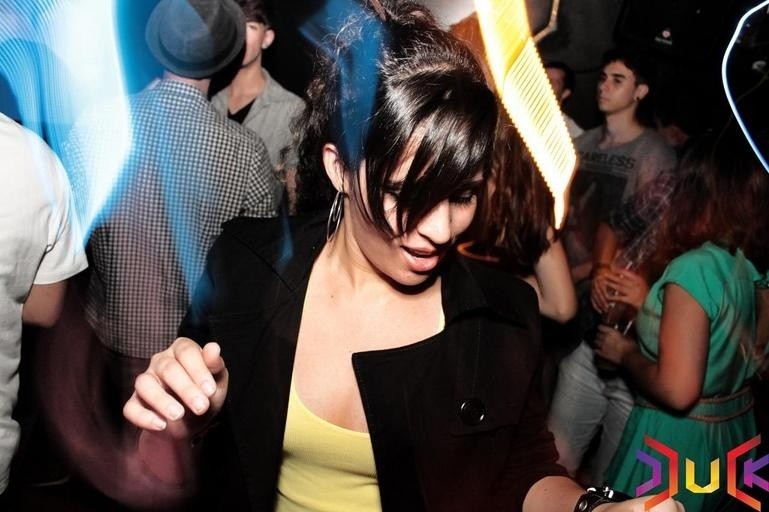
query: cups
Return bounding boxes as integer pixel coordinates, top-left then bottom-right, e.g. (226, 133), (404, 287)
(591, 300), (634, 372)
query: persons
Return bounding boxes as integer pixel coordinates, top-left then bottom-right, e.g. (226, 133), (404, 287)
(1, 114), (90, 511)
(83, 1), (769, 512)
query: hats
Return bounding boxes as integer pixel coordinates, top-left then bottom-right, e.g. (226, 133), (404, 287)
(146, 1), (247, 79)
